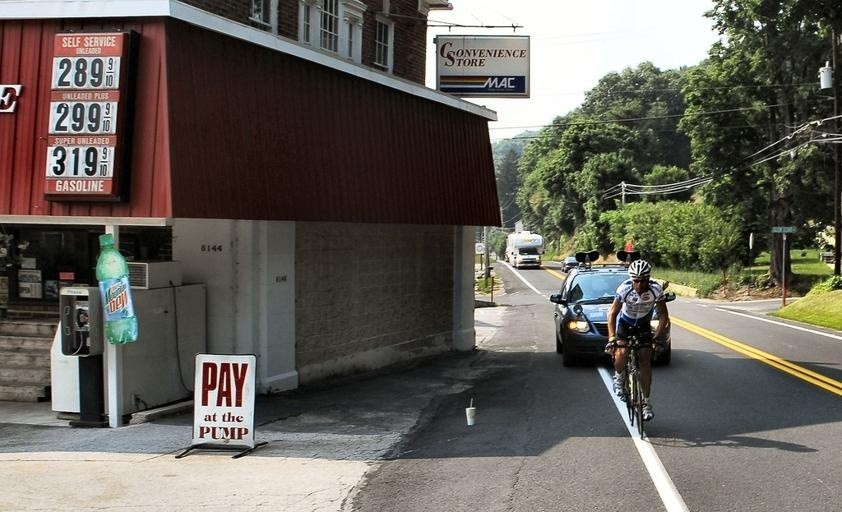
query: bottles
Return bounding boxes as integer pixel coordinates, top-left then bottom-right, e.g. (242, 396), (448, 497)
(96, 233), (138, 346)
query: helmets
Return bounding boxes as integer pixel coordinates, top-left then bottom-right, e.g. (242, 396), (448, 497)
(628, 259), (651, 278)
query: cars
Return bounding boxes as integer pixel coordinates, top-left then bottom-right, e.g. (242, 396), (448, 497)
(516, 245), (541, 268)
(551, 262), (675, 366)
(561, 256), (579, 272)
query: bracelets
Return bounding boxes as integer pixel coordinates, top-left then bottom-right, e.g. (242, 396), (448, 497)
(608, 336), (616, 342)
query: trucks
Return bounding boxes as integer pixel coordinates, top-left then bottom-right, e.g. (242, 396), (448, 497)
(505, 231), (545, 266)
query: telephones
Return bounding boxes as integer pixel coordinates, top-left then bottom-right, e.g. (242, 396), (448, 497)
(72, 301), (90, 331)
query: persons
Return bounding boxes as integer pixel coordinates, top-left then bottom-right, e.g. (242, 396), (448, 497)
(603, 258), (669, 421)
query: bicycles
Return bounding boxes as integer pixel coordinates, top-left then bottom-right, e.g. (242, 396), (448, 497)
(606, 334), (653, 439)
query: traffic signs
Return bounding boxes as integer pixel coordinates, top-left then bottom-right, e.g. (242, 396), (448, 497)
(772, 226), (796, 234)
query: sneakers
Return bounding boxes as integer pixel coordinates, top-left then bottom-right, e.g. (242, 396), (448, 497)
(641, 397), (654, 420)
(614, 369), (626, 396)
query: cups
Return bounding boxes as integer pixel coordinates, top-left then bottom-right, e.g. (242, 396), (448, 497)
(465, 408), (476, 426)
